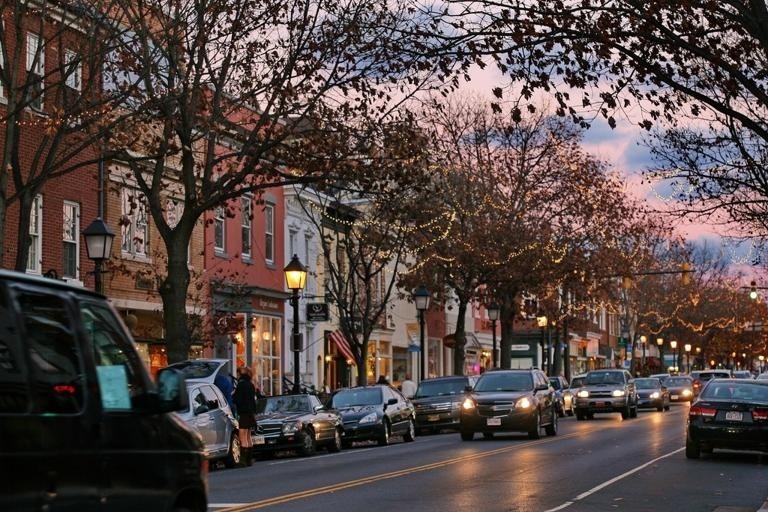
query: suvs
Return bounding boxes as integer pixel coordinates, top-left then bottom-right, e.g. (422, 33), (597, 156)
(0, 268), (210, 511)
(466, 369), (555, 444)
(573, 368), (637, 420)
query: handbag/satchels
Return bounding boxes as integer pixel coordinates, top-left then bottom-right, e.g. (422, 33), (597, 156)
(254, 389), (267, 414)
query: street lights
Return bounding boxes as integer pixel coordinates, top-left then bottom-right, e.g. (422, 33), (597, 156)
(655, 333), (666, 375)
(280, 250), (309, 393)
(411, 278), (432, 383)
(747, 287), (768, 299)
(638, 334), (646, 375)
(533, 307), (547, 373)
(669, 337), (678, 375)
(485, 294), (500, 371)
(683, 341), (692, 374)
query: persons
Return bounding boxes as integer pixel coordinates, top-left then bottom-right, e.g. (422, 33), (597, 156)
(399, 370), (418, 401)
(377, 375), (390, 384)
(231, 364), (259, 467)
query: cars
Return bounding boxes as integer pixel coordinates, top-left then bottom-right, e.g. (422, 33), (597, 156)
(632, 377), (670, 412)
(664, 376), (700, 406)
(648, 374), (670, 382)
(250, 390), (347, 456)
(156, 355), (242, 468)
(689, 367), (767, 392)
(412, 373), (475, 434)
(682, 375), (766, 460)
(546, 372), (585, 414)
(328, 385), (417, 446)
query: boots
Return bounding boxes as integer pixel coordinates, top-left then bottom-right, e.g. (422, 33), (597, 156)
(234, 446), (253, 468)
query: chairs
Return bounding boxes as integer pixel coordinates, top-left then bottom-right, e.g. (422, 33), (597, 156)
(717, 387), (731, 395)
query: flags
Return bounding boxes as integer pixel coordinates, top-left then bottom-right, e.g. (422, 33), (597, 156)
(330, 327), (358, 367)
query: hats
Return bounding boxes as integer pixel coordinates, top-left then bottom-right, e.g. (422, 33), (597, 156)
(238, 367), (252, 378)
